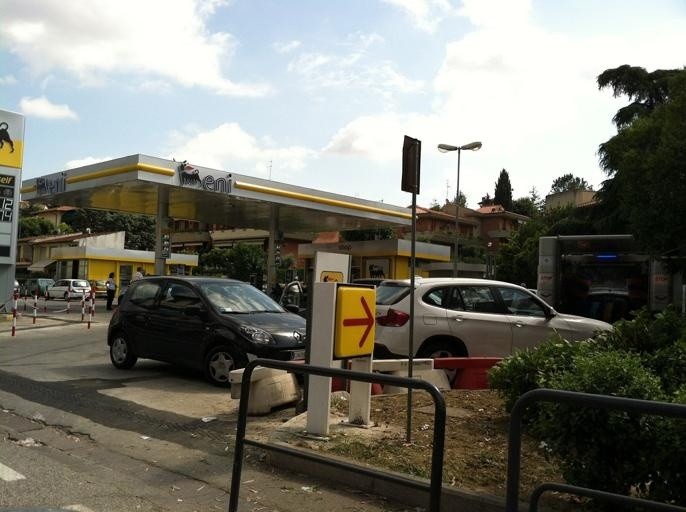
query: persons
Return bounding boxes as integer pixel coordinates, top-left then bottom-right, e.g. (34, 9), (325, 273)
(105, 272), (117, 311)
(135, 266), (144, 281)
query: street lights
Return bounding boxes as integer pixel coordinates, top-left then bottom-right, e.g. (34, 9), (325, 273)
(485, 241), (494, 277)
(434, 138), (485, 278)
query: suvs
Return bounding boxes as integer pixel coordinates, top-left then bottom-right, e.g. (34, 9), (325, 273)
(373, 275), (625, 387)
(105, 275), (314, 388)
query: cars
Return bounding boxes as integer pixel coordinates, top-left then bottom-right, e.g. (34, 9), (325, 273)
(499, 287), (538, 301)
(13, 276), (108, 302)
(349, 276), (388, 290)
